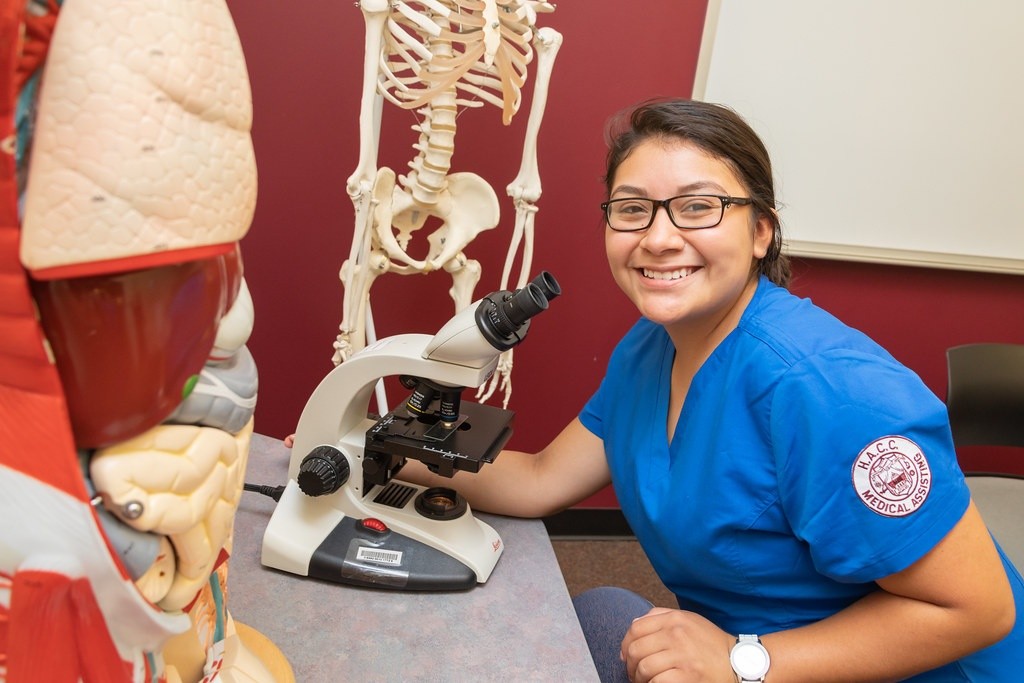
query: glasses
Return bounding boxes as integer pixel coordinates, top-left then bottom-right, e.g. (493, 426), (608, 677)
(601, 194), (751, 232)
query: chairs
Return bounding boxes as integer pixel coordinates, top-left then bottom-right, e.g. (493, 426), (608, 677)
(942, 344), (1024, 582)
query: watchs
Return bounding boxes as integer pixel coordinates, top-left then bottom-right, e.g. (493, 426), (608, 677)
(730, 632), (769, 683)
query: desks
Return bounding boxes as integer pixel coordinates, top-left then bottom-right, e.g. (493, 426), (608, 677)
(230, 490), (601, 682)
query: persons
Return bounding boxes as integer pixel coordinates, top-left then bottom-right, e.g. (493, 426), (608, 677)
(284, 96), (1024, 683)
